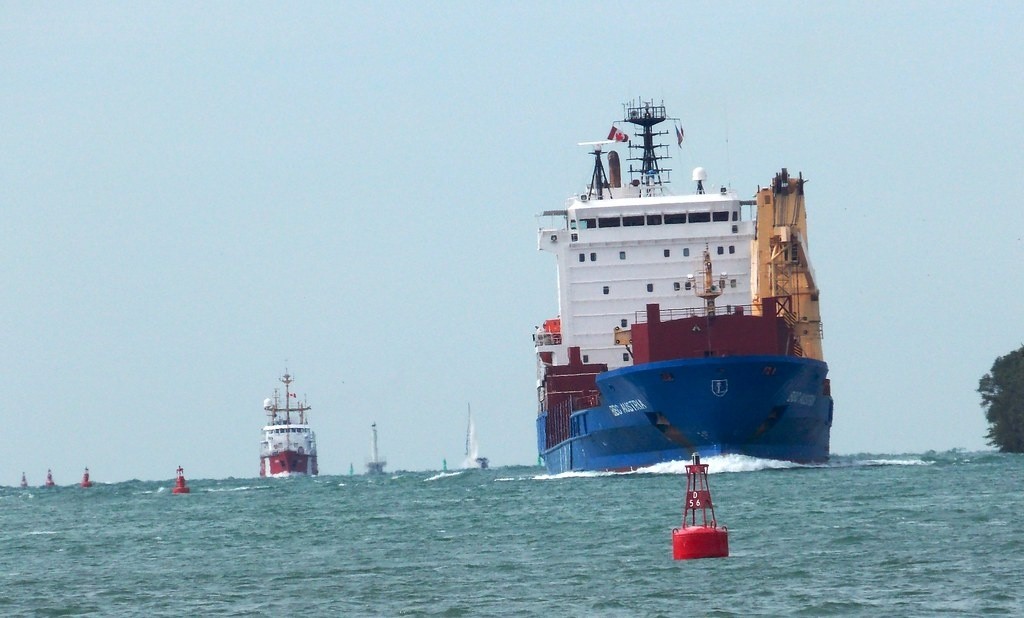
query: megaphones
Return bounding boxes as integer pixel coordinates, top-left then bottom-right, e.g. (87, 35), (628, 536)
(631, 111), (637, 117)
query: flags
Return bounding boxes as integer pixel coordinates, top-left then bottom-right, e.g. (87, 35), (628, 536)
(675, 125), (683, 148)
(607, 125), (628, 142)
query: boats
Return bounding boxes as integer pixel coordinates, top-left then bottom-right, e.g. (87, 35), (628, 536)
(20, 471), (28, 487)
(44, 466), (55, 488)
(80, 465), (92, 488)
(672, 451), (729, 560)
(172, 464), (190, 494)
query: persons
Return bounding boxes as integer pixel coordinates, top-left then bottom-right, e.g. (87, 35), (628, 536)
(535, 326), (541, 341)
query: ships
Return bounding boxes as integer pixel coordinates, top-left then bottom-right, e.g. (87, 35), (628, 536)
(259, 366), (319, 478)
(531, 95), (835, 480)
